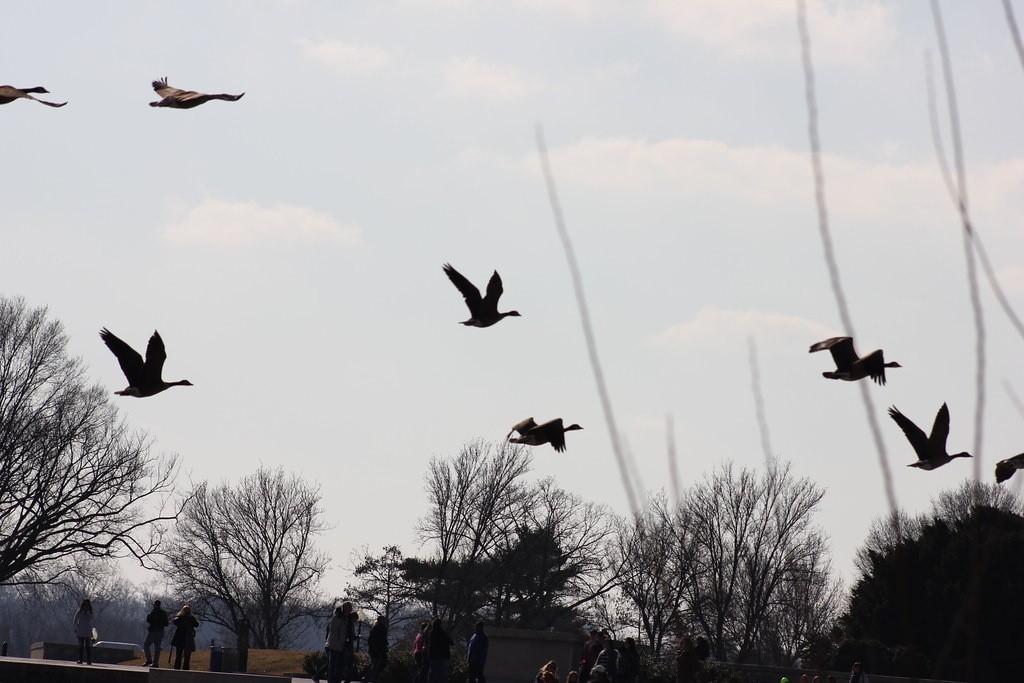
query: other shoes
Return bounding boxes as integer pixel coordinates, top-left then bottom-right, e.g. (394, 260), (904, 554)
(87, 661), (90, 665)
(142, 661), (152, 667)
(77, 660), (82, 664)
(150, 664), (159, 668)
(312, 675), (320, 683)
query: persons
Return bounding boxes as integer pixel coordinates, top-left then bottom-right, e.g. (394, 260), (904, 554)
(362, 616), (389, 683)
(170, 605), (199, 670)
(73, 599), (93, 665)
(849, 662), (869, 683)
(536, 629), (640, 683)
(412, 622), (427, 683)
(142, 600), (169, 668)
(421, 624), (431, 683)
(312, 602), (362, 683)
(467, 622), (489, 683)
(675, 637), (699, 683)
(430, 619), (454, 683)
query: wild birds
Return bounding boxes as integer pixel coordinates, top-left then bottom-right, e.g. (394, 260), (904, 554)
(0, 85), (69, 107)
(99, 326), (195, 398)
(507, 417), (584, 453)
(886, 401), (974, 471)
(441, 262), (521, 328)
(994, 452), (1024, 485)
(149, 76), (245, 110)
(808, 337), (902, 387)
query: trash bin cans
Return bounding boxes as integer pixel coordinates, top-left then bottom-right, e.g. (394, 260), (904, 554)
(210, 646), (241, 673)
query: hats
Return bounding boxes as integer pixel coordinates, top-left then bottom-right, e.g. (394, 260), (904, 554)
(591, 665), (606, 672)
